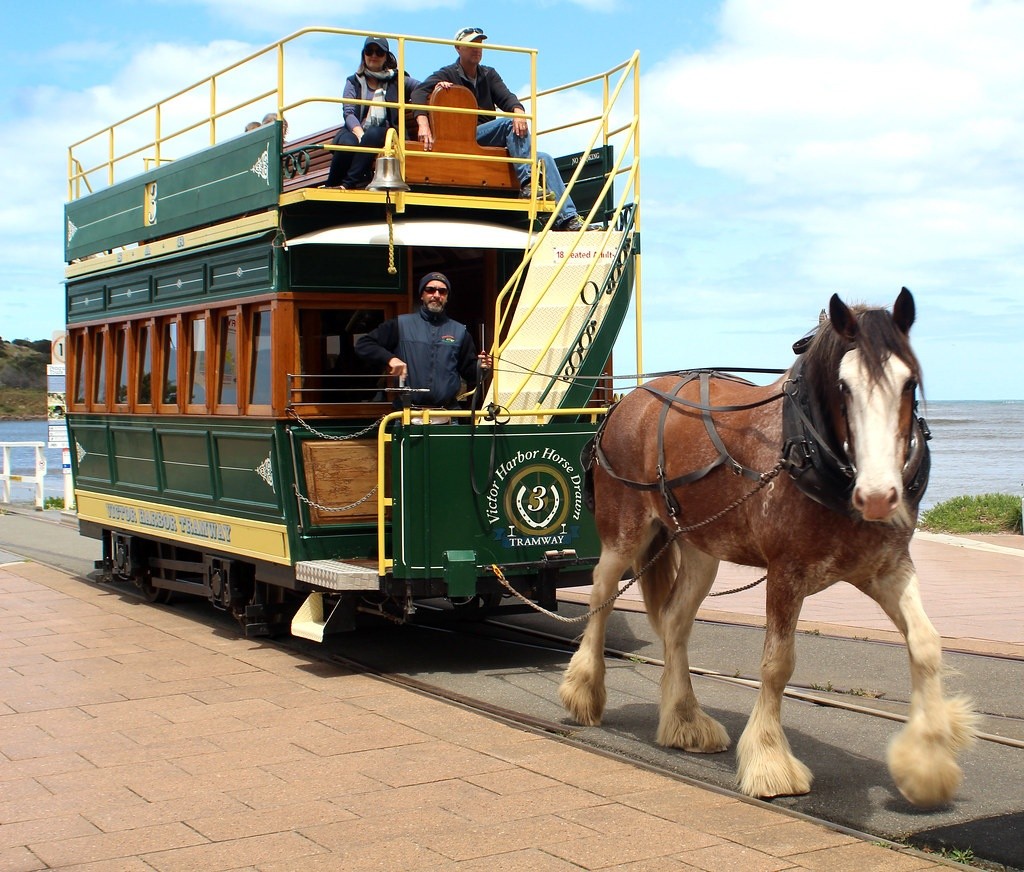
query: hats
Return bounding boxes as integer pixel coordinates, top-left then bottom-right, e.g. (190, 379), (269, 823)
(419, 271), (451, 299)
(364, 35), (389, 51)
(455, 27), (487, 42)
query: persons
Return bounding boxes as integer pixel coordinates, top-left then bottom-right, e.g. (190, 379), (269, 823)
(318, 36), (418, 190)
(240, 113), (290, 146)
(357, 272), (492, 427)
(413, 27), (603, 231)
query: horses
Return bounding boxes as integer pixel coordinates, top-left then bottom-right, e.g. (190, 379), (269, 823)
(556, 285), (984, 810)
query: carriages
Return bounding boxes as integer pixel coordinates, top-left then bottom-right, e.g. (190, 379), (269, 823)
(60, 26), (983, 810)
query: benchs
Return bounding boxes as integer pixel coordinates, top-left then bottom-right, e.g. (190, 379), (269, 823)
(280, 83), (521, 191)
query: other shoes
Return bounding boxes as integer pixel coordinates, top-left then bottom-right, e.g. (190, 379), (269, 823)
(318, 184), (342, 189)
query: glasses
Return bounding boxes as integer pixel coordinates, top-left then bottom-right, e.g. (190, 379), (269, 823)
(364, 47), (387, 57)
(423, 286), (449, 295)
(455, 28), (483, 47)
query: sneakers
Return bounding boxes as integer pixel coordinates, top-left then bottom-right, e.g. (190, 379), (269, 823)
(518, 181), (555, 201)
(564, 215), (603, 231)
